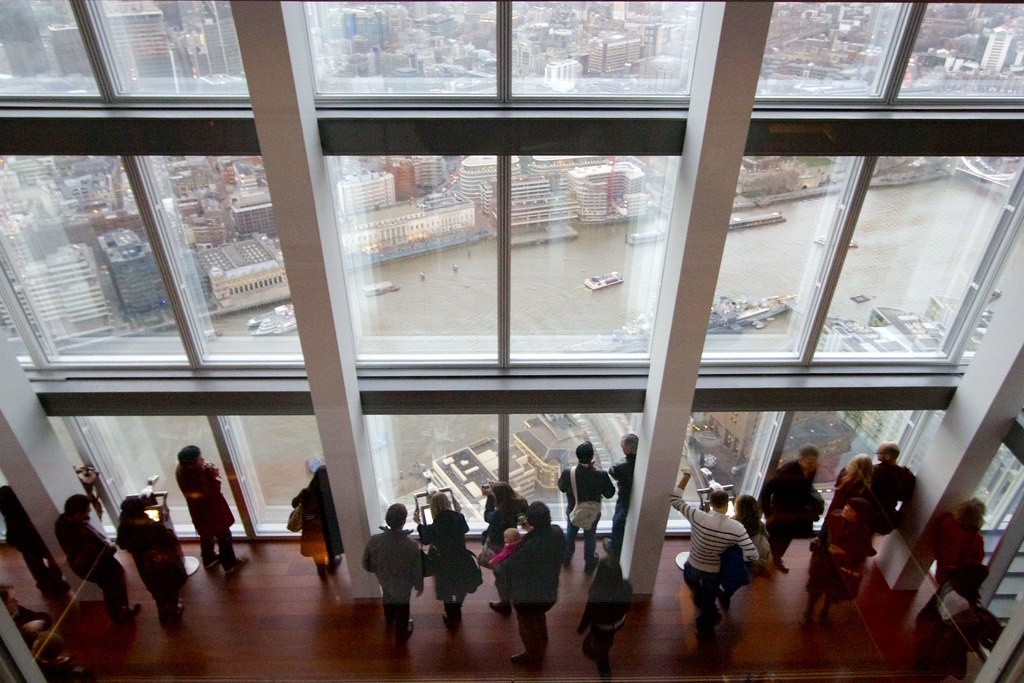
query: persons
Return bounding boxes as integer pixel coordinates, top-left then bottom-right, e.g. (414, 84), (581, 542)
(115, 497), (189, 624)
(555, 440), (616, 567)
(909, 496), (999, 683)
(801, 442), (918, 627)
(757, 445), (825, 579)
(669, 467), (774, 645)
(291, 464), (345, 579)
(601, 432), (645, 557)
(0, 484), (98, 683)
(576, 555), (634, 683)
(54, 494), (142, 626)
(175, 445), (251, 576)
(361, 480), (565, 664)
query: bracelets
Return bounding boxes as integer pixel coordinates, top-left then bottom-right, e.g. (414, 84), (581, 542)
(683, 472), (691, 478)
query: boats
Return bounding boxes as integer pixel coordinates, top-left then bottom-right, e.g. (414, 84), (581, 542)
(817, 237), (860, 248)
(728, 213), (787, 230)
(246, 303), (296, 336)
(706, 290), (791, 333)
(627, 229), (665, 245)
(584, 271), (625, 291)
(363, 281), (400, 297)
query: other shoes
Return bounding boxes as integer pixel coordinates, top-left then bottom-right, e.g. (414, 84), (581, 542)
(224, 556), (247, 574)
(489, 601), (511, 615)
(694, 614), (721, 643)
(800, 610), (811, 625)
(396, 619), (414, 646)
(159, 598), (184, 624)
(443, 611), (458, 630)
(774, 560), (789, 573)
(41, 580), (71, 598)
(111, 603), (133, 625)
(203, 554), (220, 569)
(819, 613), (825, 624)
(318, 570), (327, 581)
(584, 552), (599, 575)
(510, 650), (544, 669)
(602, 537), (620, 562)
(326, 554), (342, 575)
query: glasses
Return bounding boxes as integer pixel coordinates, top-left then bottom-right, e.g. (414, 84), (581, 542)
(875, 452), (887, 454)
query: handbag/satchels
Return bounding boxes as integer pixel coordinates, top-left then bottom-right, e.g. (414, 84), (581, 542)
(582, 629), (612, 660)
(287, 488), (307, 533)
(477, 535), (503, 570)
(418, 524), (441, 577)
(568, 500), (601, 532)
